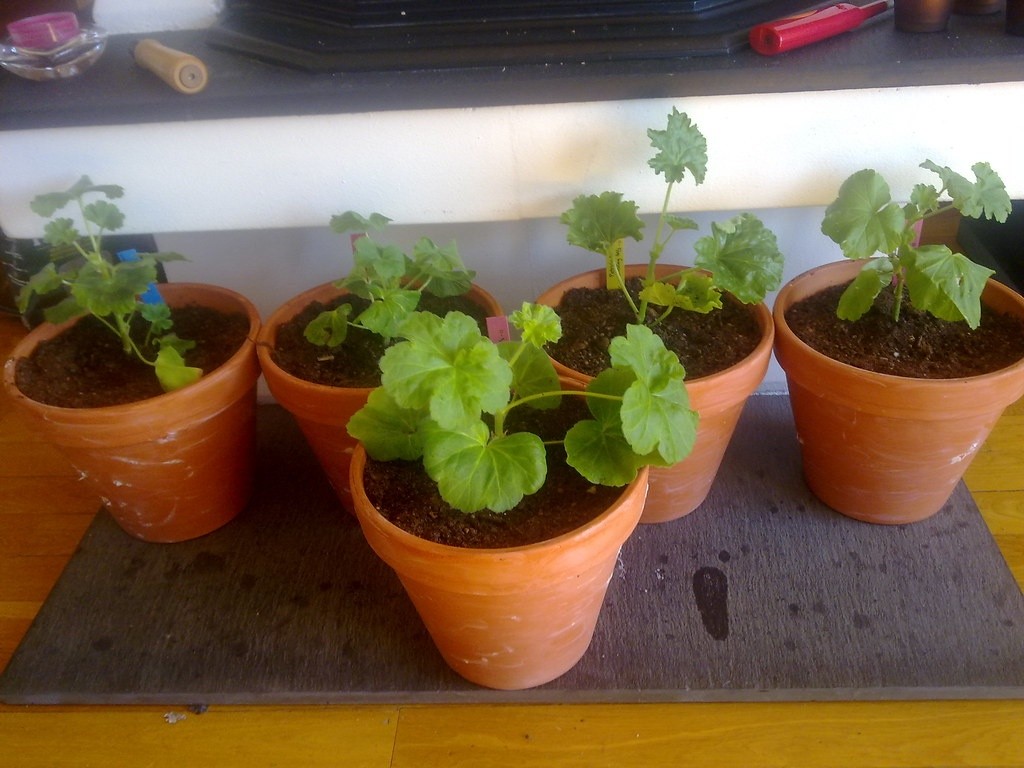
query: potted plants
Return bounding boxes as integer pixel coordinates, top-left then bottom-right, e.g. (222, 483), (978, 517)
(4, 174), (256, 544)
(258, 209), (504, 519)
(348, 304), (652, 688)
(524, 106), (783, 524)
(774, 156), (1023, 528)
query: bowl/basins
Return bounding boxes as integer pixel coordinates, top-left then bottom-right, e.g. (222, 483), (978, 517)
(0, 25), (110, 82)
